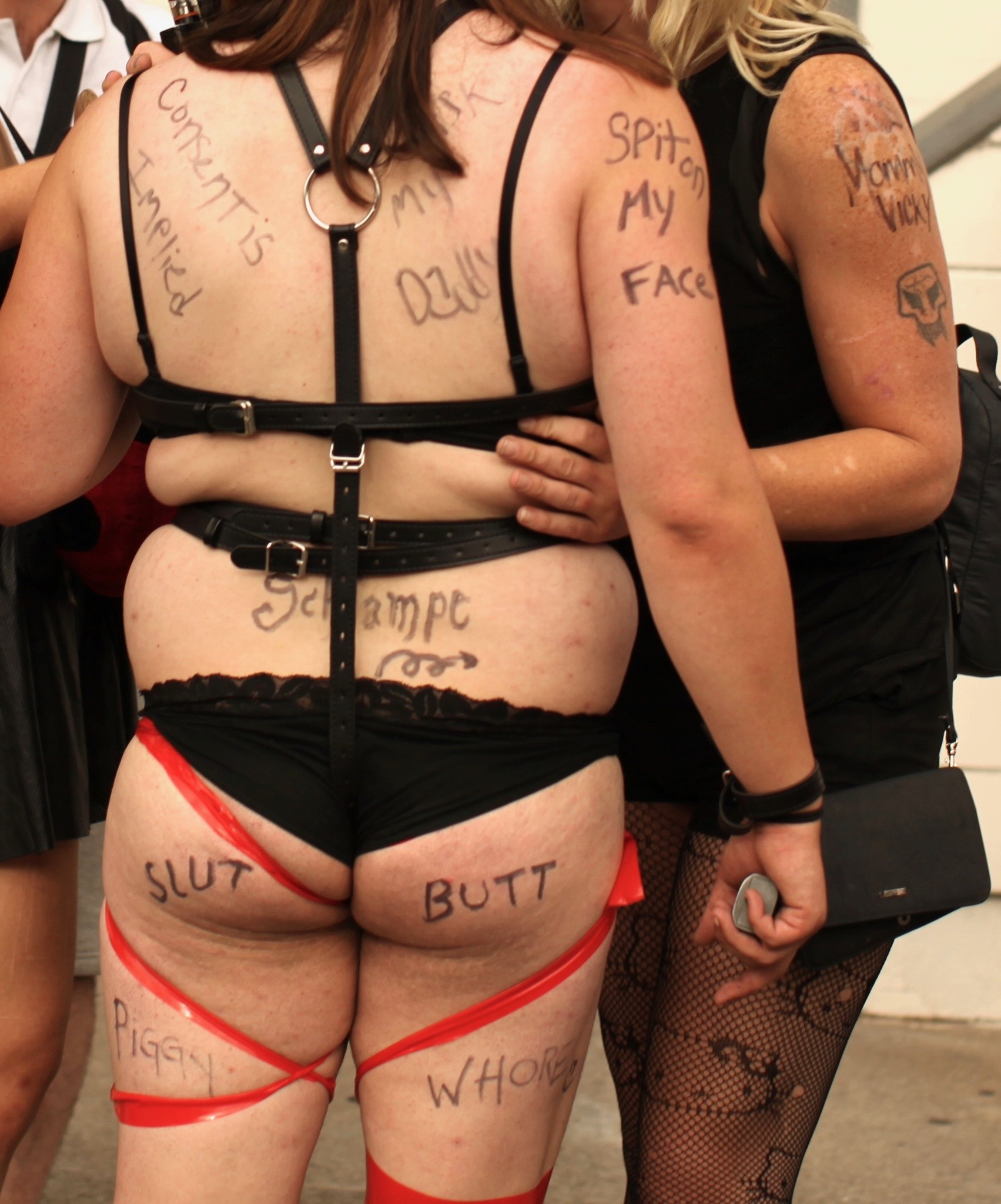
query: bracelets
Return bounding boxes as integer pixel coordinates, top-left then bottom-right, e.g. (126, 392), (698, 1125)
(720, 757), (825, 836)
(753, 806), (824, 825)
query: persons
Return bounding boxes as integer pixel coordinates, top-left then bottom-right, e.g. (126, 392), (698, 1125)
(0, 0), (1001, 1202)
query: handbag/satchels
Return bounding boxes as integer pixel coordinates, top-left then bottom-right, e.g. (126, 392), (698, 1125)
(797, 765), (992, 968)
(933, 324), (1001, 678)
